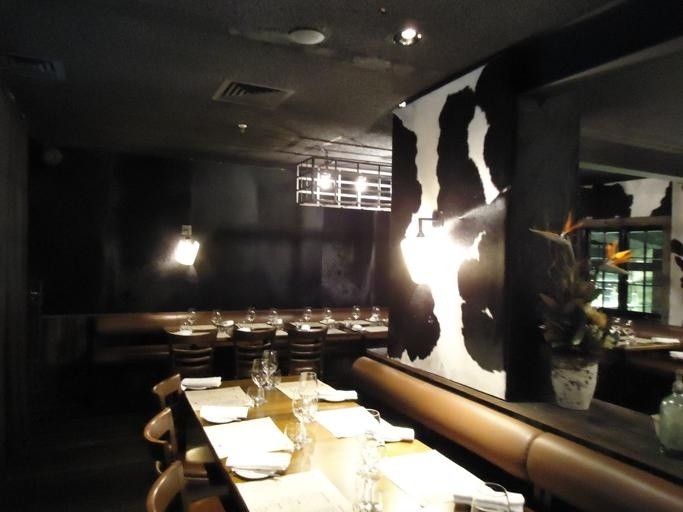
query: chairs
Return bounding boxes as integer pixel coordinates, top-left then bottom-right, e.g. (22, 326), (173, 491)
(144, 465), (243, 511)
(153, 373), (182, 407)
(142, 408), (227, 492)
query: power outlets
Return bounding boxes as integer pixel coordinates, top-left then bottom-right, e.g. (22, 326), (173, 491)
(181, 225), (192, 235)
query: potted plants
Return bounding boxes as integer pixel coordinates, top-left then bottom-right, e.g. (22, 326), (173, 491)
(530, 209), (631, 412)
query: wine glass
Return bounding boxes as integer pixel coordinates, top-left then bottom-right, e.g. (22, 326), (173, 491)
(186, 309), (199, 325)
(210, 311), (222, 325)
(251, 349), (278, 402)
(354, 409), (384, 464)
(269, 311), (277, 326)
(303, 309), (312, 322)
(291, 372), (318, 444)
(352, 306), (360, 322)
(323, 308), (332, 324)
(246, 310), (256, 325)
(372, 306), (380, 321)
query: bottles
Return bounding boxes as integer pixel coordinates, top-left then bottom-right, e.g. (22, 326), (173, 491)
(658, 378), (683, 463)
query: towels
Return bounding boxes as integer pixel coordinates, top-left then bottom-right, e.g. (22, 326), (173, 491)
(365, 424), (414, 442)
(179, 374), (222, 389)
(226, 450), (292, 471)
(200, 404), (249, 419)
(452, 489), (525, 512)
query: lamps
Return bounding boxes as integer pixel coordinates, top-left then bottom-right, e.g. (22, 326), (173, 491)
(320, 149), (333, 189)
(356, 172), (368, 194)
(174, 229), (201, 265)
(288, 27), (325, 46)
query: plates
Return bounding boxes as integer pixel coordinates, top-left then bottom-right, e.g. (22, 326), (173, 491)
(204, 417), (234, 424)
(234, 469), (271, 480)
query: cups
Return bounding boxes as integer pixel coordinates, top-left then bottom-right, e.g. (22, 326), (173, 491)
(353, 468), (391, 512)
(284, 422), (307, 451)
(247, 385), (265, 407)
